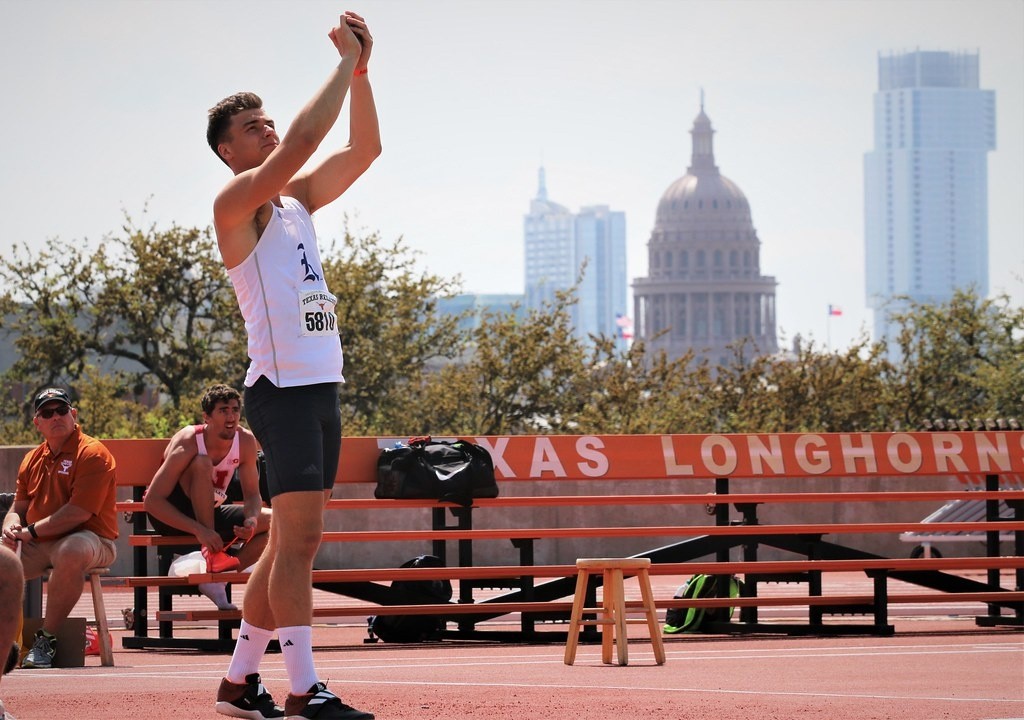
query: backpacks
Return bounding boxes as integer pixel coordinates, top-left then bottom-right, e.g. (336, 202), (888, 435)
(664, 572), (745, 634)
(364, 555), (453, 644)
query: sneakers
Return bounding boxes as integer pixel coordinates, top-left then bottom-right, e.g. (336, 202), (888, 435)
(201, 526), (254, 573)
(284, 678), (375, 720)
(84, 626), (113, 655)
(216, 672), (285, 720)
(22, 630), (58, 669)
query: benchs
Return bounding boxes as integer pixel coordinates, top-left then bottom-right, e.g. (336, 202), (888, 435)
(93, 432), (1024, 651)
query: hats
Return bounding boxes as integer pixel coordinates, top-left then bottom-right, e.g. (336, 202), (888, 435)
(35, 388), (72, 412)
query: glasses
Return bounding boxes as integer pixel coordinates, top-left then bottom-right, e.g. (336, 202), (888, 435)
(36, 406), (71, 419)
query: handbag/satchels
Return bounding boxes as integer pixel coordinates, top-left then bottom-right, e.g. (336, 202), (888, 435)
(225, 450), (272, 507)
(374, 441), (499, 517)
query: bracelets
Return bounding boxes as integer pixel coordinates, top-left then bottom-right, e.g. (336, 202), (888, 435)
(353, 68), (368, 77)
(27, 523), (38, 539)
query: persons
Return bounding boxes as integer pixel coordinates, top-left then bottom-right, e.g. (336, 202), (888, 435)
(0, 545), (25, 720)
(142, 384), (273, 610)
(204, 12), (380, 720)
(0, 388), (120, 669)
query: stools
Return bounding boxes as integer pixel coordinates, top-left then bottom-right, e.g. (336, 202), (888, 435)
(44, 567), (115, 666)
(564, 557), (665, 668)
